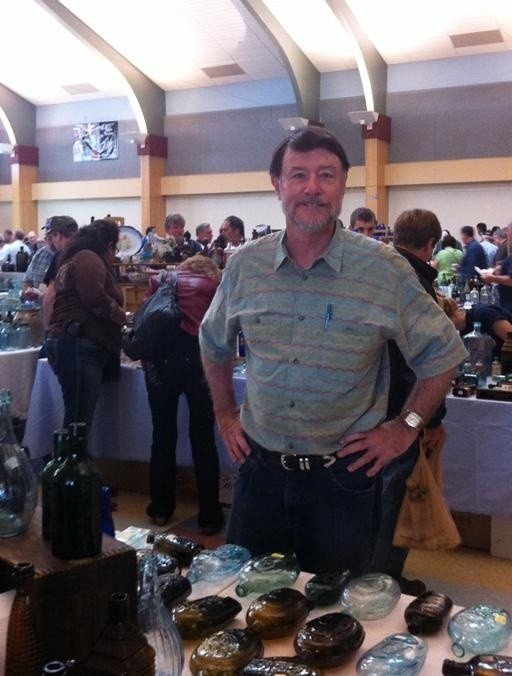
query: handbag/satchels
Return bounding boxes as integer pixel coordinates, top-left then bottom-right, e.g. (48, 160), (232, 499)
(123, 284), (181, 362)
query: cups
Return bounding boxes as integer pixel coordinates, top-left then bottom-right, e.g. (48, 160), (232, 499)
(119, 266), (128, 276)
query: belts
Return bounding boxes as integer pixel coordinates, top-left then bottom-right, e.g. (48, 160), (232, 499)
(240, 433), (359, 475)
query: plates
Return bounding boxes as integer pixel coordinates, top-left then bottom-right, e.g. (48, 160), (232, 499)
(114, 225), (145, 260)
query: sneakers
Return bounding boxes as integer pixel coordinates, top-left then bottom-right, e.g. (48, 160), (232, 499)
(201, 520), (228, 538)
(395, 576), (426, 596)
(151, 513), (172, 527)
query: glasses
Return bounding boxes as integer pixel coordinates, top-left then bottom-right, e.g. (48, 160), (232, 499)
(350, 221), (378, 234)
(104, 242), (120, 256)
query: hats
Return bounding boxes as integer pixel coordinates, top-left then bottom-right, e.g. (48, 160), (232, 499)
(41, 216), (60, 230)
(45, 216), (79, 235)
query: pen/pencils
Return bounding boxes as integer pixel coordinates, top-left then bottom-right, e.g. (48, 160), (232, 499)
(324, 303), (332, 329)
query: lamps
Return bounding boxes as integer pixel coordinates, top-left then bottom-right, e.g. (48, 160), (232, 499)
(0, 522), (512, 676)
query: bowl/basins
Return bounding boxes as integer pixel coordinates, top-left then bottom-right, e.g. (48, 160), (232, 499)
(126, 272), (159, 282)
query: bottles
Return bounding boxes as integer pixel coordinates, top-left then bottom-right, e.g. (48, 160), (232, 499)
(154, 566), (193, 601)
(252, 229), (258, 241)
(232, 549), (303, 597)
(357, 630), (428, 676)
(41, 431), (70, 542)
(372, 223), (385, 241)
(160, 230), (225, 272)
(189, 619), (265, 674)
(166, 594), (244, 635)
(290, 612), (365, 668)
(304, 568), (350, 613)
(102, 486), (115, 536)
(445, 601), (512, 657)
(52, 422), (102, 558)
(435, 273), (511, 398)
(399, 589), (451, 641)
(438, 651), (512, 676)
(0, 244), (44, 352)
(338, 572), (402, 625)
(242, 587), (310, 642)
(187, 541), (250, 585)
(266, 225), (271, 236)
(146, 533), (205, 564)
(0, 387), (38, 539)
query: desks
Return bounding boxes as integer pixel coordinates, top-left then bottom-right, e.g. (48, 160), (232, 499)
(0, 345), (43, 420)
(26, 351), (512, 558)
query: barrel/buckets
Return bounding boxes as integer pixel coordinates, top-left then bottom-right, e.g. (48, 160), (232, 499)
(461, 322), (495, 387)
